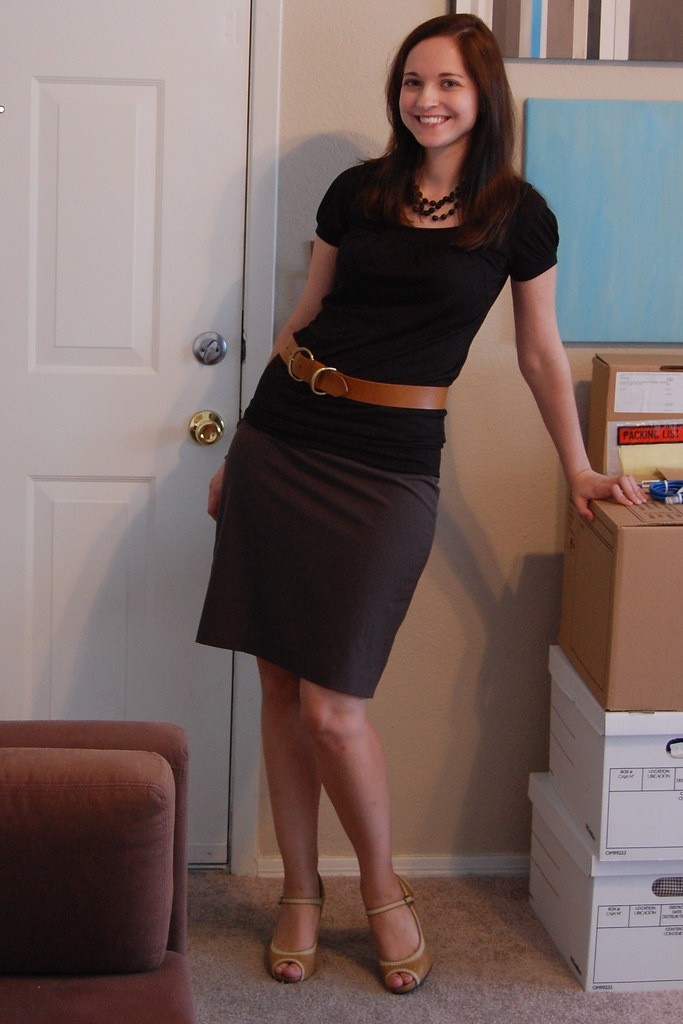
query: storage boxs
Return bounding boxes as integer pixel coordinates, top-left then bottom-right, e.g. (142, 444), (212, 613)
(528, 348), (680, 995)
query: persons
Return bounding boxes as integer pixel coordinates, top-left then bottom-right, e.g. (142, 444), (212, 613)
(195, 13), (647, 994)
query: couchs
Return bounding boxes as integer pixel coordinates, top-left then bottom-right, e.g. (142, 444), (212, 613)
(0, 719), (199, 1023)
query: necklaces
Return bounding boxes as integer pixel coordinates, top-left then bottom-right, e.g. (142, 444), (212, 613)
(409, 179), (460, 222)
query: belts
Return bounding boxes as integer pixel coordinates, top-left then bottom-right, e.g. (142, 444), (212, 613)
(279, 334), (449, 410)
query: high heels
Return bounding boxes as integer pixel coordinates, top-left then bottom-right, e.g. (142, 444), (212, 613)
(366, 872), (432, 993)
(267, 872), (325, 982)
